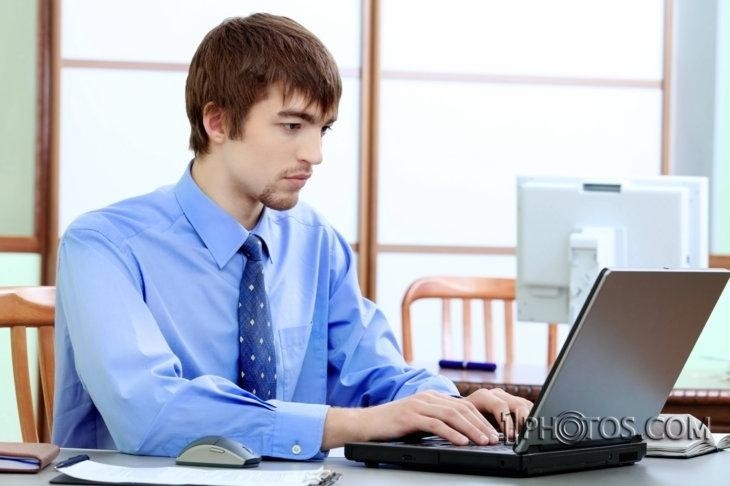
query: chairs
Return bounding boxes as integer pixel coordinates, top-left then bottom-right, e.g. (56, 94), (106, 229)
(1, 281), (66, 448)
(397, 274), (558, 371)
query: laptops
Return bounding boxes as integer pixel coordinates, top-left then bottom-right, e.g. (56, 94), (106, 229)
(344, 268), (730, 478)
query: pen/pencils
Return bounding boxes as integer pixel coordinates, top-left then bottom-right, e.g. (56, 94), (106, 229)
(439, 360), (496, 371)
(54, 455), (89, 469)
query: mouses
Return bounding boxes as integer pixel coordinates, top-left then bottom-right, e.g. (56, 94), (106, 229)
(175, 436), (261, 468)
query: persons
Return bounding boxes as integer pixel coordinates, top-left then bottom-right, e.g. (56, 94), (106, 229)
(52, 11), (536, 463)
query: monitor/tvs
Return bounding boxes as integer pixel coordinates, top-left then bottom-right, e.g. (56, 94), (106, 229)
(516, 173), (708, 325)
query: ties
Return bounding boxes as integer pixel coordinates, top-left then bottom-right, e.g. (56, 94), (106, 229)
(235, 232), (282, 402)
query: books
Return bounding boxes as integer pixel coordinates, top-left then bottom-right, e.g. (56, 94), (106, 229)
(0, 443), (61, 474)
(645, 412), (730, 459)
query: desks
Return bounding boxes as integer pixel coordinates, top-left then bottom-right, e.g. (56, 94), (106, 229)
(0, 438), (729, 486)
(424, 354), (727, 434)
